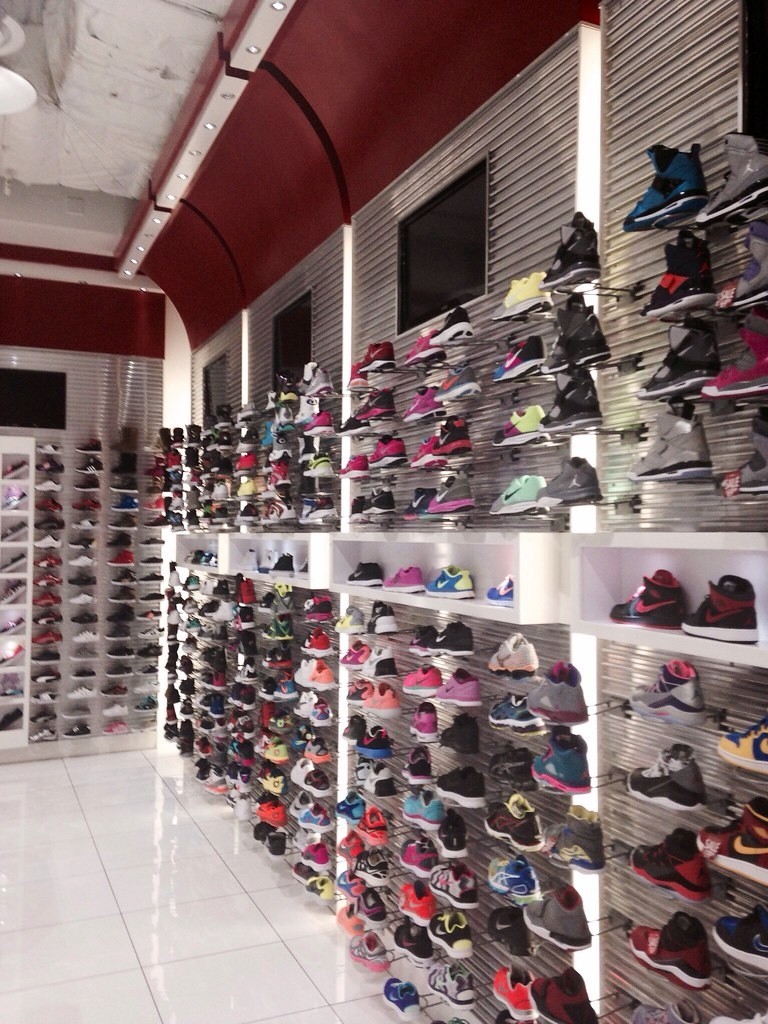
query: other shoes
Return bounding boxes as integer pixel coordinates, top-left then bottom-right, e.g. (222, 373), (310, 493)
(161, 550), (257, 823)
(202, 362), (339, 528)
(141, 424), (202, 533)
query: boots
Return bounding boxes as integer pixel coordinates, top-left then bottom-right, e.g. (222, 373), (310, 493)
(108, 425), (139, 452)
(111, 449), (137, 475)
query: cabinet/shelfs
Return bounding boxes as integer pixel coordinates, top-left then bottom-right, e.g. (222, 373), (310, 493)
(175, 531), (579, 627)
(0, 436), (35, 749)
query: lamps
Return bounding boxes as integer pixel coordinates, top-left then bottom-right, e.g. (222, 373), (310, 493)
(0, 0), (67, 113)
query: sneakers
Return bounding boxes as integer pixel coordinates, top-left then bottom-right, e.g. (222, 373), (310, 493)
(696, 132), (767, 226)
(681, 575), (759, 644)
(522, 663), (597, 729)
(537, 372), (604, 434)
(696, 798), (766, 886)
(488, 467), (549, 515)
(622, 142), (708, 231)
(531, 728), (591, 796)
(539, 303), (613, 376)
(536, 457), (605, 508)
(250, 545), (335, 907)
(521, 881), (591, 954)
(488, 271), (553, 321)
(712, 904), (767, 972)
(627, 742), (707, 812)
(715, 223), (767, 313)
(488, 405), (547, 447)
(607, 569), (682, 631)
(551, 800), (605, 874)
(339, 305), (475, 533)
(633, 997), (700, 1024)
(716, 717), (768, 775)
(710, 1012), (767, 1024)
(636, 233), (718, 318)
(633, 324), (722, 400)
(629, 828), (709, 906)
(334, 561), (546, 1024)
(492, 335), (546, 381)
(535, 213), (593, 290)
(700, 315), (767, 401)
(628, 659), (707, 724)
(2, 423), (164, 744)
(628, 912), (711, 990)
(720, 406), (767, 492)
(628, 412), (718, 484)
(529, 967), (599, 1024)
